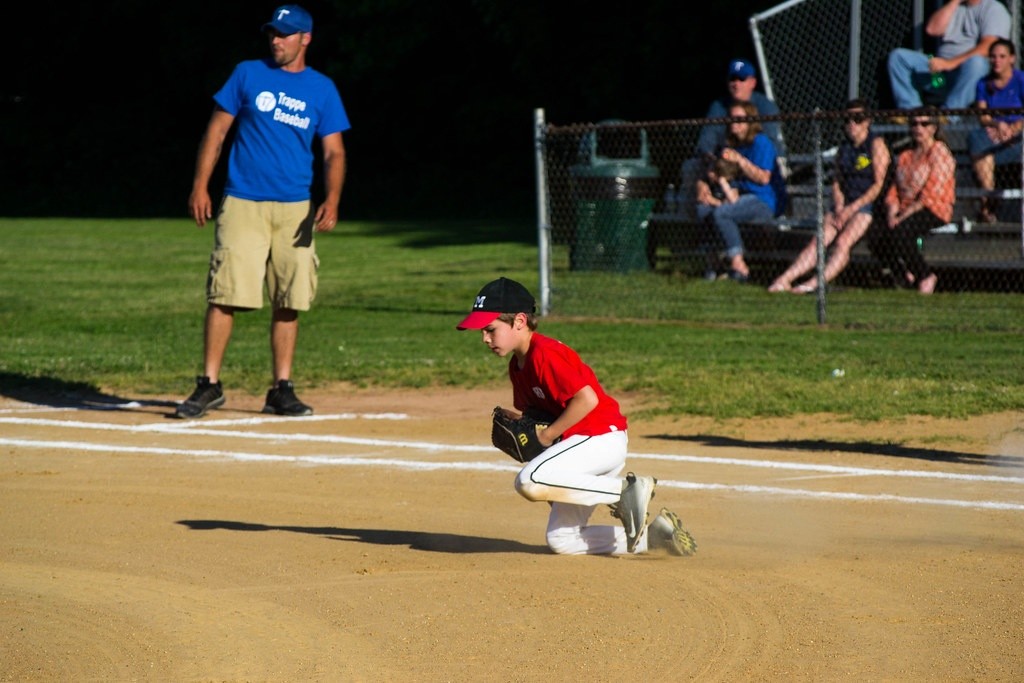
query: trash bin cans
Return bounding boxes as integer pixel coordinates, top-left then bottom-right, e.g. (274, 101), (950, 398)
(566, 118), (657, 272)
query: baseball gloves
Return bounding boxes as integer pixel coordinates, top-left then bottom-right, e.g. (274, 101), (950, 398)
(491, 406), (563, 464)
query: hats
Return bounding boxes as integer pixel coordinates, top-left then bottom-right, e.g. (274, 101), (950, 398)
(727, 59), (755, 77)
(261, 4), (313, 34)
(456, 277), (536, 330)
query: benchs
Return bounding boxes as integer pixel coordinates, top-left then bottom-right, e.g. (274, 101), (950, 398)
(652, 121), (1024, 288)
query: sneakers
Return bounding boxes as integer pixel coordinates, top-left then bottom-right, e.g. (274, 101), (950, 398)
(652, 507), (697, 556)
(610, 472), (658, 552)
(176, 375), (225, 418)
(262, 379), (313, 416)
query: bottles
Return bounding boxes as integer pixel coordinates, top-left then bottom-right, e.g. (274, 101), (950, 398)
(928, 54), (946, 87)
(665, 184), (676, 214)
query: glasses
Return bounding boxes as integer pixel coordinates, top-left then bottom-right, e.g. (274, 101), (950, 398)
(911, 121), (933, 126)
(842, 116), (864, 124)
(729, 75), (747, 81)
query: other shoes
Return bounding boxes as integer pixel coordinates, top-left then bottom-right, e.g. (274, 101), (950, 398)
(930, 105), (949, 125)
(717, 268), (751, 282)
(767, 279), (815, 294)
(887, 115), (909, 124)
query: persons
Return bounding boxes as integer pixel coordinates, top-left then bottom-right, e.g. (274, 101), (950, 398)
(174, 4), (352, 419)
(678, 61), (793, 284)
(965, 37), (1024, 224)
(766, 101), (896, 295)
(456, 276), (697, 557)
(867, 106), (956, 295)
(886, 0), (1011, 125)
